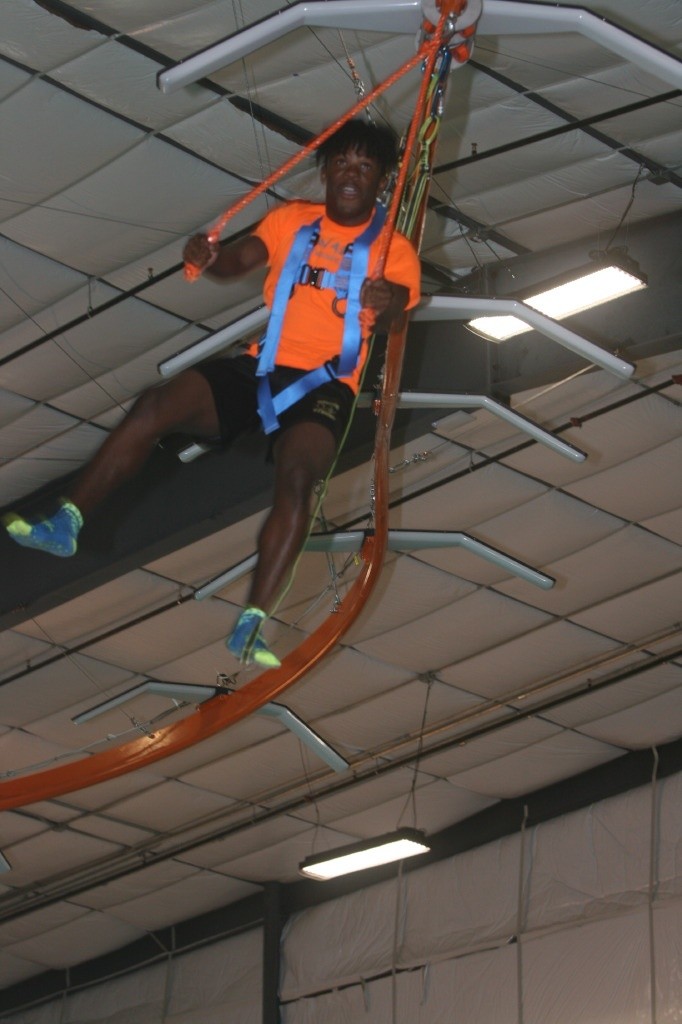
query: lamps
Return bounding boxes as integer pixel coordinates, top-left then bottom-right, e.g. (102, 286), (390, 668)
(463, 161), (651, 345)
(294, 671), (436, 881)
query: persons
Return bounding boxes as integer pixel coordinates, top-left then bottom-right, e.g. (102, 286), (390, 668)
(1, 121), (420, 669)
